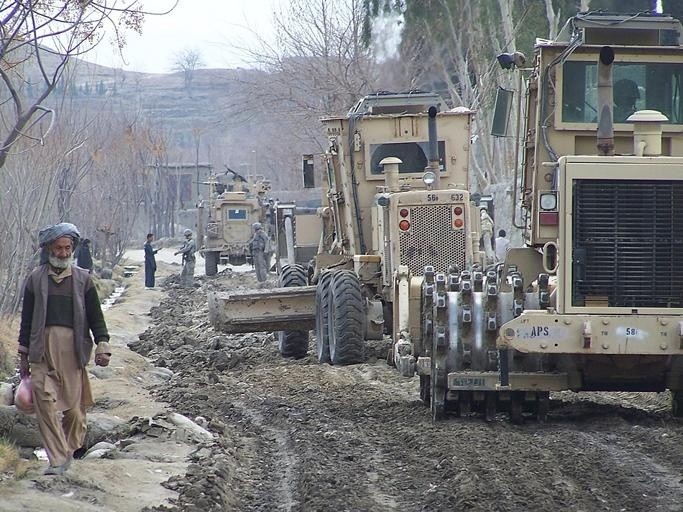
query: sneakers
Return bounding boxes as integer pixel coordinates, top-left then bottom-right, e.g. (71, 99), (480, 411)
(74, 432), (88, 458)
(44, 464), (65, 476)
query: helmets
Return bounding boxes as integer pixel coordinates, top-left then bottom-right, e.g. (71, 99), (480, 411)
(183, 229), (192, 237)
(253, 223), (261, 229)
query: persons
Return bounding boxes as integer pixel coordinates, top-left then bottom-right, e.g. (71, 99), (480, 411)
(144, 233), (163, 290)
(73, 238), (95, 275)
(590, 79), (641, 123)
(17, 222), (112, 475)
(249, 222), (270, 282)
(174, 228), (196, 287)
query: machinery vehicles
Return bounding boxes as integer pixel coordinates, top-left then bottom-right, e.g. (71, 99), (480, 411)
(192, 164), (273, 275)
(209, 89), (499, 363)
(387, 11), (683, 419)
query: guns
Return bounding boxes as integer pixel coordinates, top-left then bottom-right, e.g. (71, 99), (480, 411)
(250, 242), (256, 269)
(225, 167), (248, 183)
(180, 244), (188, 267)
(191, 181), (249, 194)
